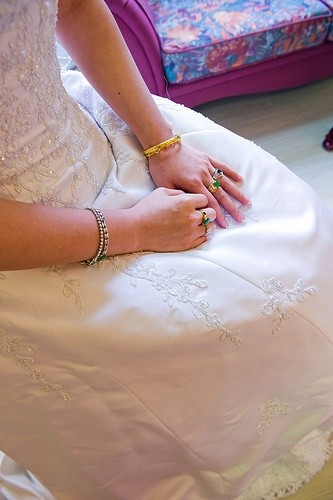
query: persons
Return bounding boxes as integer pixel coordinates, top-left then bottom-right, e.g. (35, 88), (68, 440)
(1, 0), (333, 500)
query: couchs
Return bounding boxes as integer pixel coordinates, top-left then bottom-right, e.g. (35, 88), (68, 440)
(105, 0), (333, 108)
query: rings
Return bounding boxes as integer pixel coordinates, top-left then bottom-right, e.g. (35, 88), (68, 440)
(207, 182), (221, 194)
(203, 225), (210, 238)
(212, 168), (223, 180)
(200, 209), (210, 226)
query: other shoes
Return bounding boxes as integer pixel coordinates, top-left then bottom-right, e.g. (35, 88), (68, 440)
(323, 128), (333, 150)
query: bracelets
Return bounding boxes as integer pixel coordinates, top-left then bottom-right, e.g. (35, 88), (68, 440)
(79, 207), (108, 267)
(143, 135), (182, 159)
